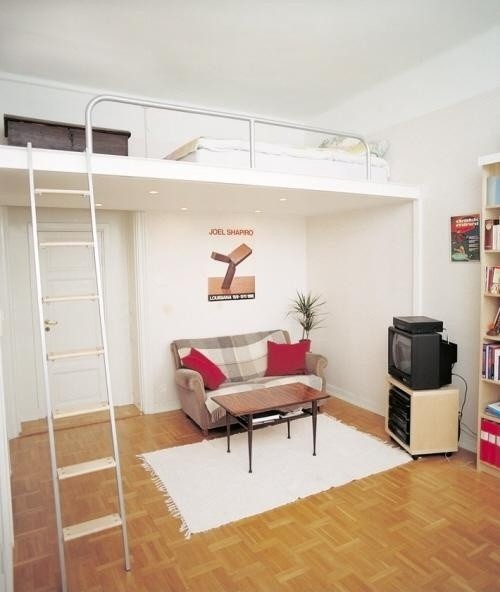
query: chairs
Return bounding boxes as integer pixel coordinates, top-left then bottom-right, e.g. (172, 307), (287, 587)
(476, 151), (500, 479)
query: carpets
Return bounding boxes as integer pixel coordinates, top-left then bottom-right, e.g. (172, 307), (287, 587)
(136, 413), (414, 541)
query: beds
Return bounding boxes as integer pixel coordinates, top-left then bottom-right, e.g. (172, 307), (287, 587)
(164, 137), (390, 181)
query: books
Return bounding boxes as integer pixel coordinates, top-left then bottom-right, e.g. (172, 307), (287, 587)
(479, 176), (500, 470)
(244, 410), (279, 423)
(239, 417), (275, 426)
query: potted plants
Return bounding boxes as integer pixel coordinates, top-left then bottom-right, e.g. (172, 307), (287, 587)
(284, 288), (328, 353)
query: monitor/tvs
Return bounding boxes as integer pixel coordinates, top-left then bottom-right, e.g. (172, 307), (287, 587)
(387, 326), (458, 390)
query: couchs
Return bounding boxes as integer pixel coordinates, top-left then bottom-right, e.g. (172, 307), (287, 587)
(170, 330), (328, 436)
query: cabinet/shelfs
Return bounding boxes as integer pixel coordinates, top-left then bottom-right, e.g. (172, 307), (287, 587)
(385, 379), (461, 457)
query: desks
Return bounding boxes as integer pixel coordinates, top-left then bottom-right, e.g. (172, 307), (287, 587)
(2, 114), (131, 155)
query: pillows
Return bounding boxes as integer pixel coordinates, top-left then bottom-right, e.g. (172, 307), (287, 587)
(320, 136), (391, 158)
(264, 339), (311, 376)
(180, 349), (226, 391)
